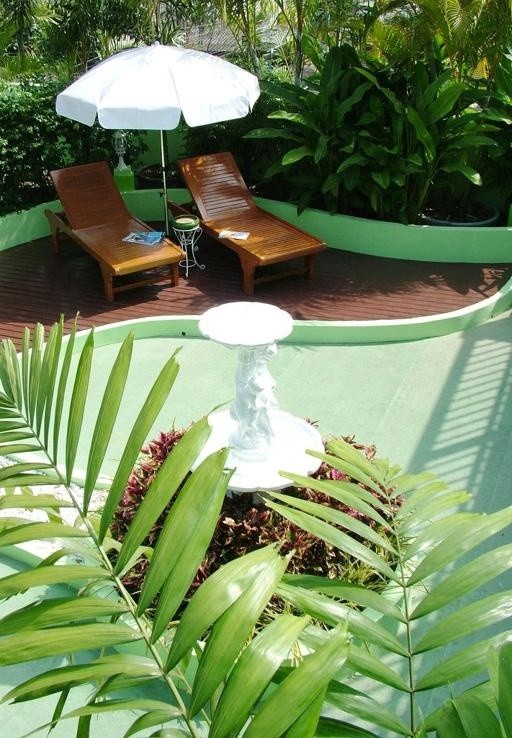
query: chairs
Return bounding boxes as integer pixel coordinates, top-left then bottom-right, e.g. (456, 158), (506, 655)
(45, 161), (188, 302)
(168, 152), (327, 296)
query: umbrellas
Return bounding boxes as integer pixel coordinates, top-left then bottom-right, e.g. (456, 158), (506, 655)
(55, 39), (263, 236)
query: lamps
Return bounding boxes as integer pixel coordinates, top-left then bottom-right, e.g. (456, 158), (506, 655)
(111, 130), (130, 173)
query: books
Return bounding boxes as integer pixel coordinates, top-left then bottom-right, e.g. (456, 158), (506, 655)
(218, 229), (251, 241)
(122, 231), (165, 246)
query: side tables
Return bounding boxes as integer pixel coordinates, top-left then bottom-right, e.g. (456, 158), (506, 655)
(172, 225), (203, 278)
